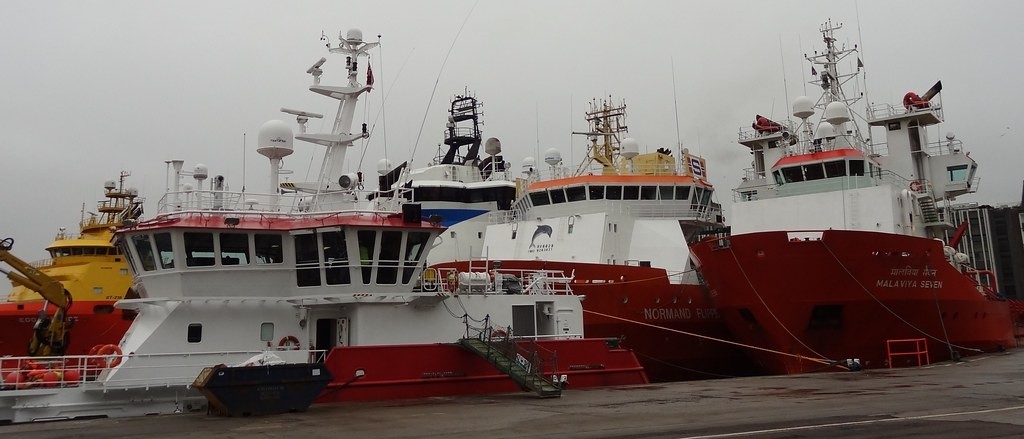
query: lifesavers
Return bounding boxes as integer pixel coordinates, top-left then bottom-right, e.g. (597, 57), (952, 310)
(490, 328), (508, 337)
(909, 180), (923, 191)
(276, 335), (300, 349)
(97, 344), (123, 367)
(89, 344), (102, 364)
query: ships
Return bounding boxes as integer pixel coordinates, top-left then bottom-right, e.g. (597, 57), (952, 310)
(0, 1), (1024, 422)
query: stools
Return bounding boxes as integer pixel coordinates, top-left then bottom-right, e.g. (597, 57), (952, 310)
(887, 338), (930, 369)
